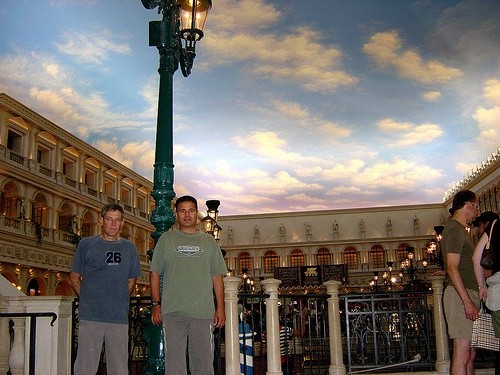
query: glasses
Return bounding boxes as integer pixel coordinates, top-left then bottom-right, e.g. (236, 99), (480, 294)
(104, 217), (122, 223)
(463, 202), (479, 209)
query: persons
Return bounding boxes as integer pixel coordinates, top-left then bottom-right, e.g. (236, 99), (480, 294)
(262, 309), (305, 375)
(431, 189), (500, 375)
(150, 195), (228, 375)
(69, 203), (141, 375)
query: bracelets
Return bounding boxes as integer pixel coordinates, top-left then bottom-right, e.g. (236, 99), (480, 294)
(478, 286), (486, 289)
(151, 301), (160, 307)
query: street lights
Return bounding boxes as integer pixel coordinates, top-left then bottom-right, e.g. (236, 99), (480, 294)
(374, 224), (445, 363)
(140, 0), (212, 375)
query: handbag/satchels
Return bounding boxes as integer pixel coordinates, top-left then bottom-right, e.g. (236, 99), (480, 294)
(471, 301), (500, 351)
(480, 219), (497, 270)
